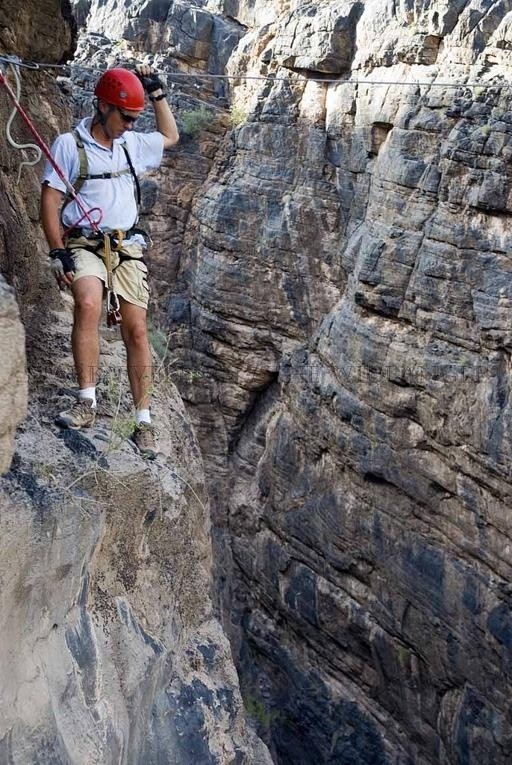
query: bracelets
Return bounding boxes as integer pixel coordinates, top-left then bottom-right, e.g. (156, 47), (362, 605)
(149, 94), (168, 102)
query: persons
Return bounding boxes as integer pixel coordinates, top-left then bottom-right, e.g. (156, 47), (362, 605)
(39, 65), (179, 461)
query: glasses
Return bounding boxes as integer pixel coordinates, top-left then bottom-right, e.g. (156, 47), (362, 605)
(116, 106), (139, 123)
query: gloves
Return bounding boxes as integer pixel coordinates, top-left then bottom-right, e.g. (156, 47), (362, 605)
(48, 248), (76, 275)
(134, 68), (162, 94)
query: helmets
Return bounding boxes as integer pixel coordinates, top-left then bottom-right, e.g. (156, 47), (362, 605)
(93, 68), (145, 111)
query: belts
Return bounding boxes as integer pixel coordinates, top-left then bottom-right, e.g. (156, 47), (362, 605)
(67, 225), (149, 240)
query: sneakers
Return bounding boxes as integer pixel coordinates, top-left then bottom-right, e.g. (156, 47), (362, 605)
(54, 397), (98, 432)
(130, 419), (159, 462)
